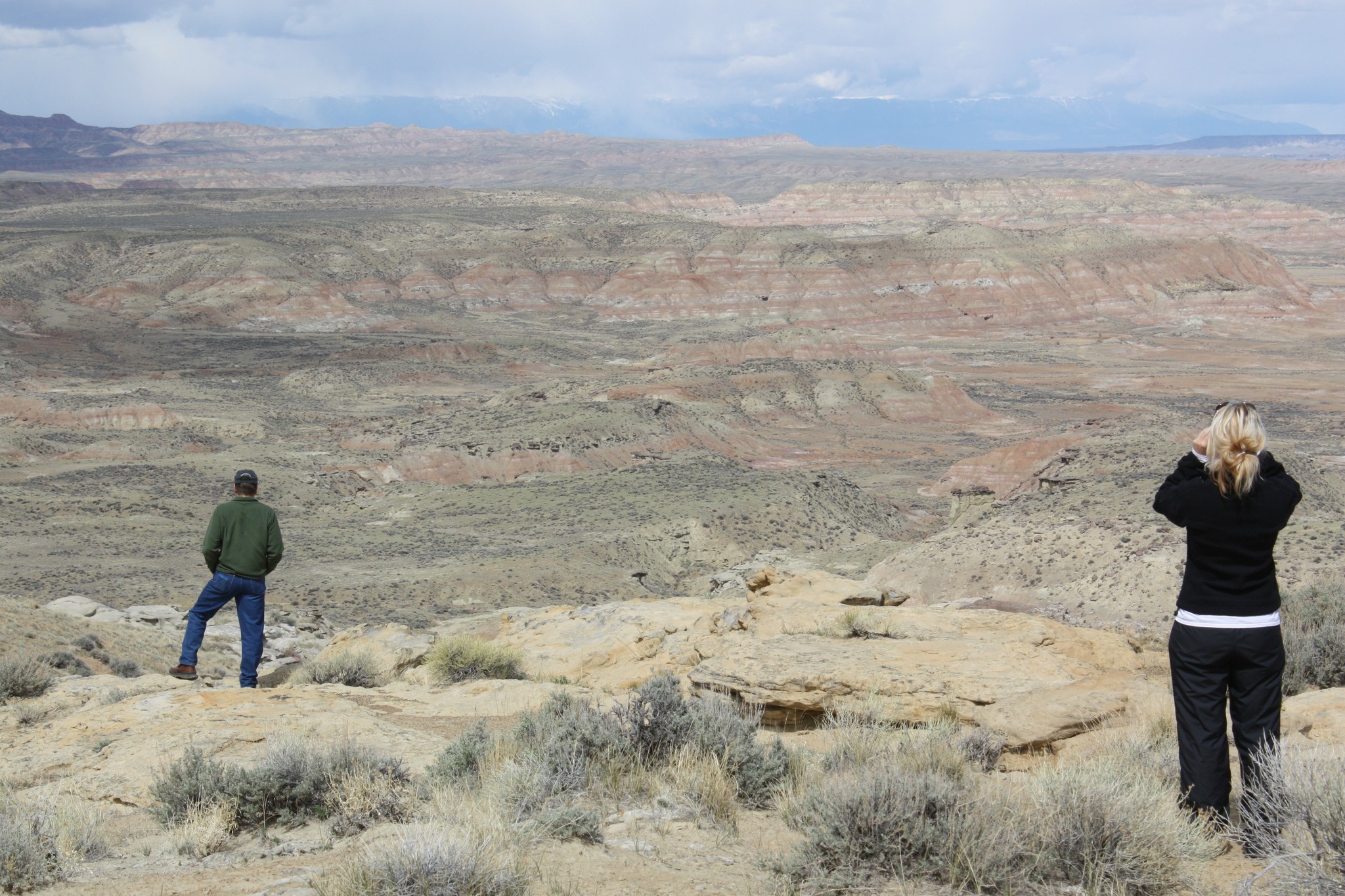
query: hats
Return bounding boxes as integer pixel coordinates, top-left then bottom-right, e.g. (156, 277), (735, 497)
(234, 470), (259, 485)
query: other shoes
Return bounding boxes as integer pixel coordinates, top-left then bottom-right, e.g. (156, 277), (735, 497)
(1240, 824), (1280, 857)
(170, 664), (197, 679)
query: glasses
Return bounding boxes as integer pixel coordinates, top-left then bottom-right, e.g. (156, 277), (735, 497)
(1215, 401), (1256, 413)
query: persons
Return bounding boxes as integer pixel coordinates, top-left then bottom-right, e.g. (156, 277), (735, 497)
(1152, 401), (1303, 859)
(170, 469), (284, 687)
